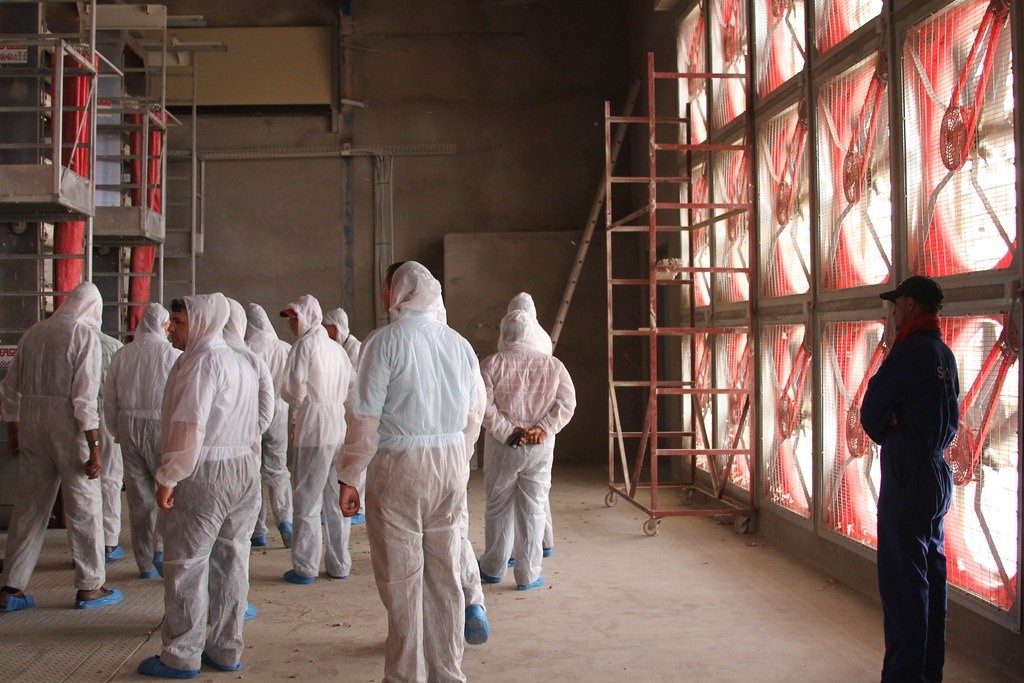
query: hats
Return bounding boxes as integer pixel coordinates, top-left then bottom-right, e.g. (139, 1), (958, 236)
(280, 309), (297, 318)
(879, 276), (944, 304)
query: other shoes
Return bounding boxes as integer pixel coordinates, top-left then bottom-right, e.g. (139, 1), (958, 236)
(466, 604), (488, 644)
(106, 546), (126, 560)
(201, 651), (242, 671)
(326, 570), (349, 579)
(477, 559), (501, 582)
(543, 548), (552, 557)
(508, 558), (516, 567)
(0, 590), (34, 609)
(141, 552), (164, 579)
(284, 569), (314, 583)
(136, 654), (200, 678)
(517, 576), (544, 590)
(351, 515), (365, 524)
(76, 587), (121, 606)
(243, 603), (257, 621)
(279, 521), (293, 548)
(251, 536), (266, 546)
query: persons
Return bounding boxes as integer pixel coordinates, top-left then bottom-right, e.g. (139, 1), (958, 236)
(383, 260), (493, 642)
(241, 302), (297, 549)
(279, 293), (355, 585)
(492, 292), (555, 565)
(476, 310), (577, 593)
(1, 282), (125, 611)
(104, 301), (184, 581)
(83, 329), (132, 562)
(320, 308), (366, 524)
(136, 297), (264, 675)
(860, 275), (962, 680)
(334, 262), (470, 683)
(219, 294), (274, 621)
